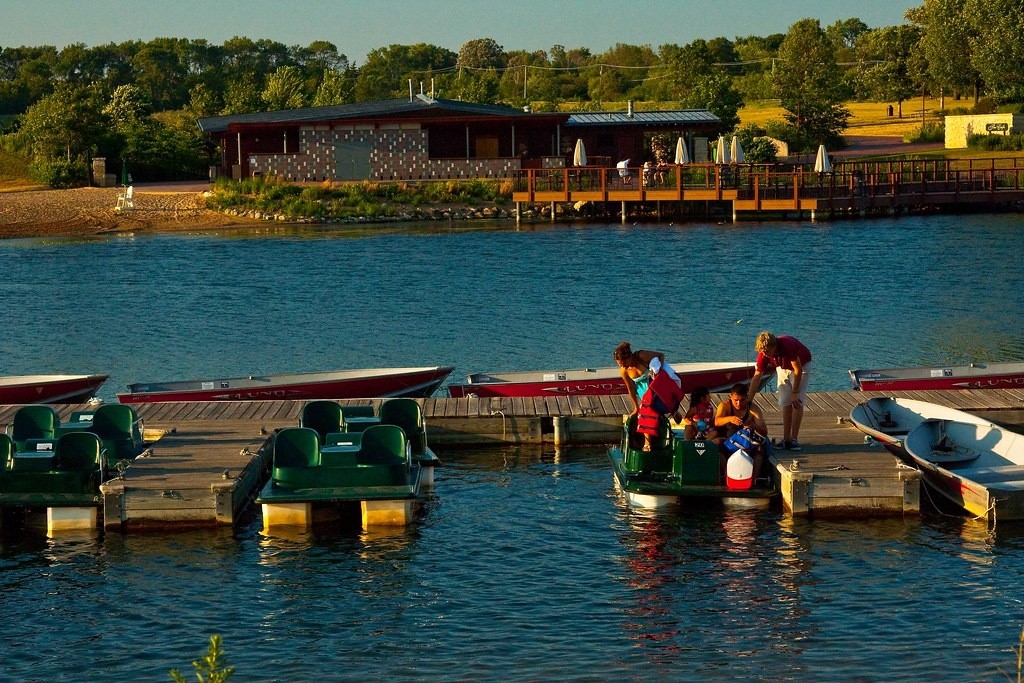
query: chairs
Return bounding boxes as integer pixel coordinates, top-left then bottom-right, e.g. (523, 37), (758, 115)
(620, 413), (767, 486)
(113, 185), (136, 210)
(647, 167), (694, 186)
(253, 398), (444, 505)
(0, 403), (143, 506)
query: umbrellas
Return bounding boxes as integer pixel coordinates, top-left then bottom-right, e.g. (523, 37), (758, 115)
(731, 136), (744, 186)
(675, 137), (689, 190)
(716, 136), (731, 189)
(573, 138), (587, 188)
(814, 145), (830, 198)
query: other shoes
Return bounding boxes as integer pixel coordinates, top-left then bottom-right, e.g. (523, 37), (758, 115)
(673, 410), (682, 424)
(643, 444), (651, 452)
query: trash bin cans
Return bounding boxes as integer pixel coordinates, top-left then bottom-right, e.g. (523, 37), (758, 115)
(849, 171), (864, 196)
(209, 166), (217, 182)
(252, 170), (263, 181)
(715, 164), (730, 188)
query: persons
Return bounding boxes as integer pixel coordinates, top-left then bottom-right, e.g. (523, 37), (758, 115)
(747, 332), (812, 451)
(714, 384), (769, 437)
(612, 342), (682, 425)
(616, 157), (632, 184)
(643, 157), (670, 185)
(684, 387), (721, 441)
(565, 150), (573, 175)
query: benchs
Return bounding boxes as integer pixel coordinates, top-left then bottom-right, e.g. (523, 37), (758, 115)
(947, 464), (1024, 476)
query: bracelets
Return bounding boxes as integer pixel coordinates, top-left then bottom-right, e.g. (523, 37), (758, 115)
(792, 391), (799, 393)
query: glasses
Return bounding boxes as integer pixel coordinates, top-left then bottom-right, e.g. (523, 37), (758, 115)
(614, 349), (626, 355)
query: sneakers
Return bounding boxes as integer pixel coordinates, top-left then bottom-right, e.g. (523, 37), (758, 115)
(791, 440), (801, 450)
(774, 438), (791, 448)
